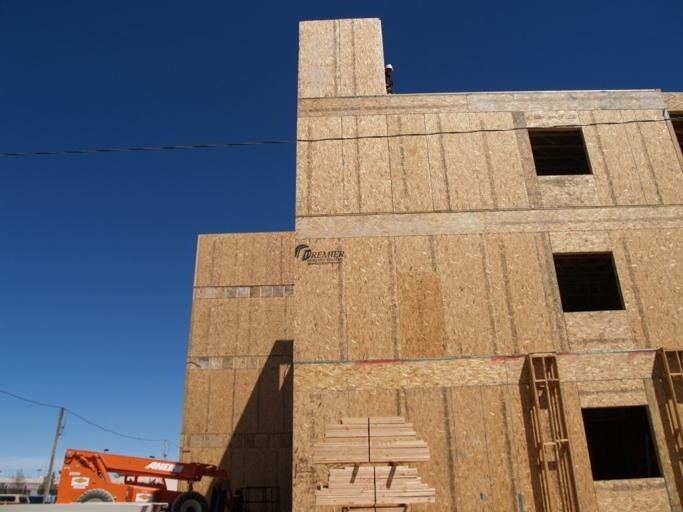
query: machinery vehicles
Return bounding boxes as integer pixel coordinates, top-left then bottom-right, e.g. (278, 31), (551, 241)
(54, 447), (240, 511)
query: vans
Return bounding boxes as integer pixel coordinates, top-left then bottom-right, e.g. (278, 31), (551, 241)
(0, 493), (57, 505)
